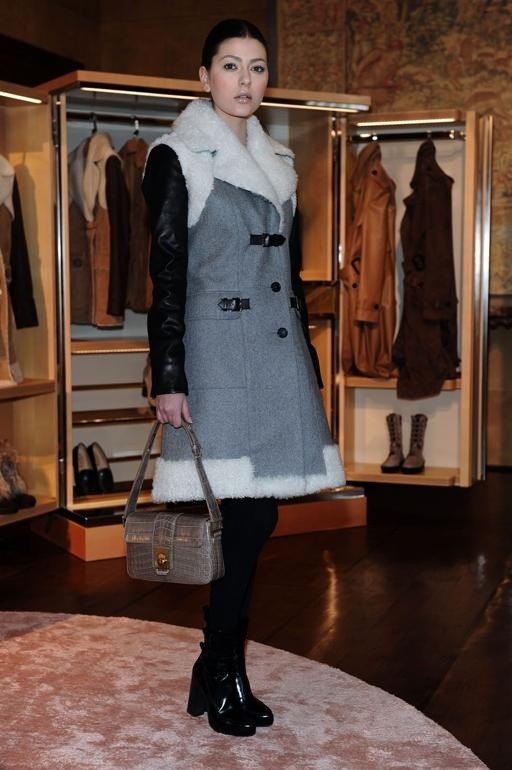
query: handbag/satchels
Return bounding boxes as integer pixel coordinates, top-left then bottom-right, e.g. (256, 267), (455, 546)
(122, 418), (228, 586)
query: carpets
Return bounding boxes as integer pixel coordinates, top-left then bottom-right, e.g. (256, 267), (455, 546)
(2, 608), (493, 770)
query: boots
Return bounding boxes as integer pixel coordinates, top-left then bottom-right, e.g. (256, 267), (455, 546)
(1, 446), (36, 515)
(402, 414), (428, 474)
(188, 604), (274, 735)
(381, 413), (405, 473)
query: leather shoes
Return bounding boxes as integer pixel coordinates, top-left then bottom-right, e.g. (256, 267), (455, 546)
(73, 442), (114, 494)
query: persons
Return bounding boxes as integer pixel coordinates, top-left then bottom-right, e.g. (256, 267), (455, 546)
(119, 22), (351, 740)
